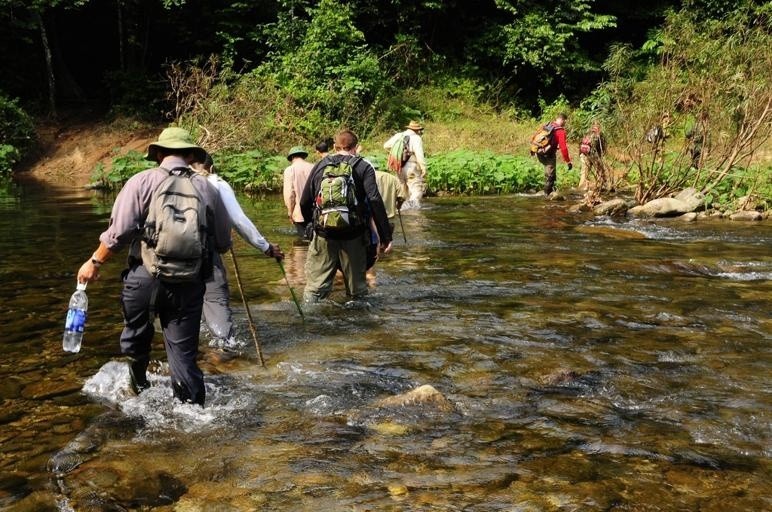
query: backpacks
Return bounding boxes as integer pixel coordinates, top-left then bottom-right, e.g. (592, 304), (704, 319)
(138, 167), (214, 285)
(390, 133), (412, 169)
(310, 159), (367, 239)
(528, 123), (557, 157)
(579, 131), (598, 157)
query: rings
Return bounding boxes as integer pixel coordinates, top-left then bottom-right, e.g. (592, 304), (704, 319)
(280, 250), (284, 257)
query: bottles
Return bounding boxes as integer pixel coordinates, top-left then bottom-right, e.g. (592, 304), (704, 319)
(62, 280), (89, 353)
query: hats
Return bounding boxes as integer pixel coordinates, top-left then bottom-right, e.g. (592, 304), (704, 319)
(403, 120), (424, 131)
(143, 126), (207, 165)
(364, 156), (381, 168)
(287, 146), (309, 161)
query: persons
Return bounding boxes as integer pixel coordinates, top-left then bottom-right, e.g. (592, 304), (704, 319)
(365, 155), (406, 253)
(316, 142), (326, 158)
(77, 127), (233, 408)
(299, 131), (393, 302)
(384, 121), (426, 211)
(579, 126), (605, 190)
(337, 232), (379, 276)
(188, 154), (286, 353)
(531, 113), (572, 195)
(283, 146), (314, 239)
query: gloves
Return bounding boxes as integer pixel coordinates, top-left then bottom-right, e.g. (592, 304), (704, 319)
(567, 162), (573, 171)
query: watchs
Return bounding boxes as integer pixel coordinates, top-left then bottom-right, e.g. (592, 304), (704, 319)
(89, 253), (104, 269)
